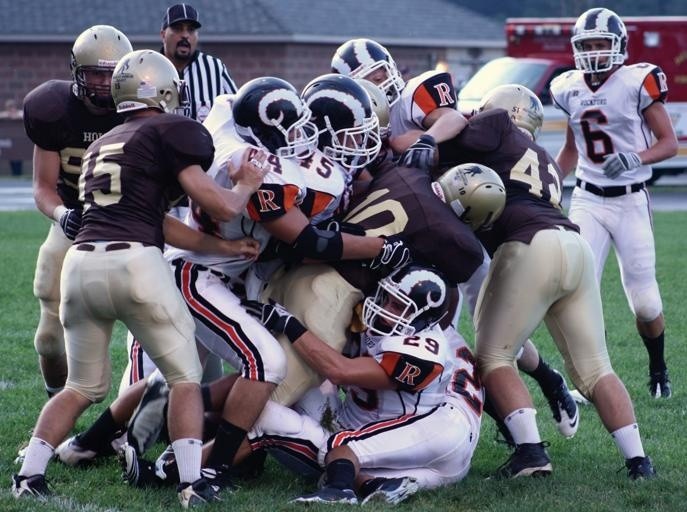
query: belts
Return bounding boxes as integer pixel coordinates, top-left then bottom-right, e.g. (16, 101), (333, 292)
(574, 176), (646, 198)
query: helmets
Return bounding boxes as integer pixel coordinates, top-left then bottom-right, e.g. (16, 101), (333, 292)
(358, 262), (452, 339)
(568, 7), (631, 75)
(68, 23), (184, 116)
(230, 37), (406, 173)
(430, 82), (546, 242)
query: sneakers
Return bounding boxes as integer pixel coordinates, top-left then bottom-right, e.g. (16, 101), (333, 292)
(623, 454), (657, 482)
(178, 477), (224, 509)
(539, 368), (580, 438)
(201, 464), (241, 493)
(288, 485), (358, 508)
(119, 442), (151, 489)
(494, 441), (554, 482)
(53, 433), (100, 466)
(647, 365), (672, 400)
(157, 444), (177, 482)
(10, 473), (56, 501)
(359, 475), (419, 508)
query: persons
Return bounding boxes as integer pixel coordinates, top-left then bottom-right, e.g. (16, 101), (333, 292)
(152, 3), (243, 126)
(549, 7), (679, 408)
(12, 24), (654, 509)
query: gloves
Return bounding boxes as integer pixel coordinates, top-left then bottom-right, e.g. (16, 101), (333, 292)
(361, 232), (423, 279)
(600, 151), (644, 181)
(238, 294), (308, 345)
(58, 206), (85, 242)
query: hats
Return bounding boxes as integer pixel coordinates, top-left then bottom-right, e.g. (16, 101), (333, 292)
(160, 1), (203, 30)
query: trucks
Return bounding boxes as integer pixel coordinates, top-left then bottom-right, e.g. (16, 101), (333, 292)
(457, 16), (686, 186)
